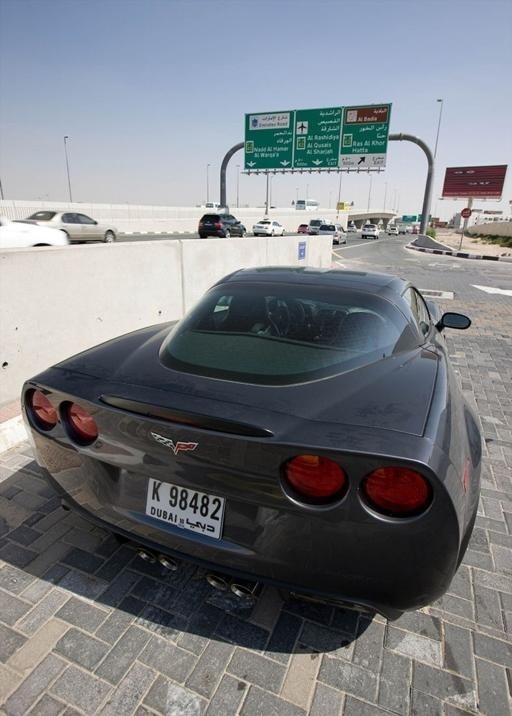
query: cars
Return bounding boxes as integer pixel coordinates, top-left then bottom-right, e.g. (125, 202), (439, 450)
(0, 211), (118, 248)
(198, 213), (246, 238)
(348, 224), (357, 232)
(388, 225), (420, 236)
(252, 218), (286, 237)
(205, 203), (214, 207)
(361, 224), (379, 239)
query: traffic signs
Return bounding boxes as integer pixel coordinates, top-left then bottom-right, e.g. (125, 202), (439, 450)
(244, 101), (392, 176)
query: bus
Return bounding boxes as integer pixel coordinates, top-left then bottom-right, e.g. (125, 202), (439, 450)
(297, 200), (319, 210)
(336, 200), (354, 211)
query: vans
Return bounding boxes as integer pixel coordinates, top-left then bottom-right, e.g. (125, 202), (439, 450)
(298, 219), (347, 245)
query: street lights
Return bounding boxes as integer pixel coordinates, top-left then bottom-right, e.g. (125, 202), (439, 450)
(433, 98), (443, 159)
(367, 174), (372, 211)
(206, 164), (212, 208)
(63, 135), (72, 202)
(236, 163), (240, 208)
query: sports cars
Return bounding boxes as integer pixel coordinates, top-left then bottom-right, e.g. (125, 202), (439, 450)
(20, 266), (480, 622)
